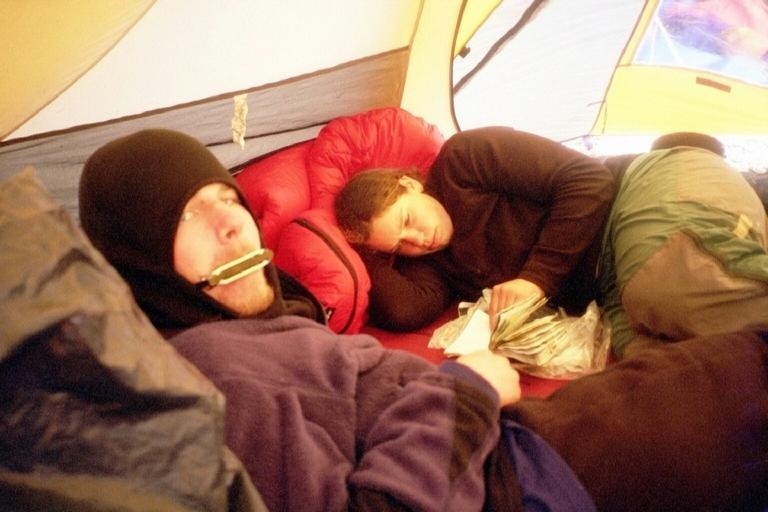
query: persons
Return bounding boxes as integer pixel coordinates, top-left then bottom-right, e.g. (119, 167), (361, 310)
(76, 127), (768, 512)
(334, 123), (768, 359)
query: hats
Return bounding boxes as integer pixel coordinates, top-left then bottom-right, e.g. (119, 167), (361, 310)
(79, 130), (287, 330)
